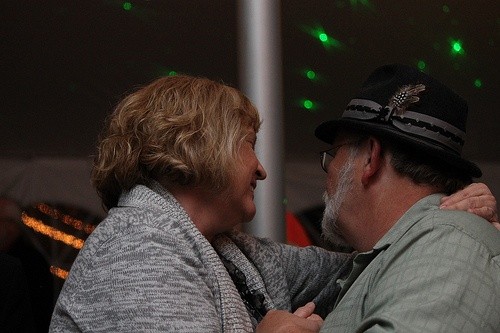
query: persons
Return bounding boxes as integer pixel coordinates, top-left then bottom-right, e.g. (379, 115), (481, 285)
(48, 75), (500, 333)
(0, 196), (54, 333)
(257, 63), (500, 333)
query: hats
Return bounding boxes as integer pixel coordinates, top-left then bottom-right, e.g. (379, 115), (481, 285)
(313, 64), (483, 179)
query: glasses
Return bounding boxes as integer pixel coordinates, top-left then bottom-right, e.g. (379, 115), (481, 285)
(320, 143), (352, 173)
